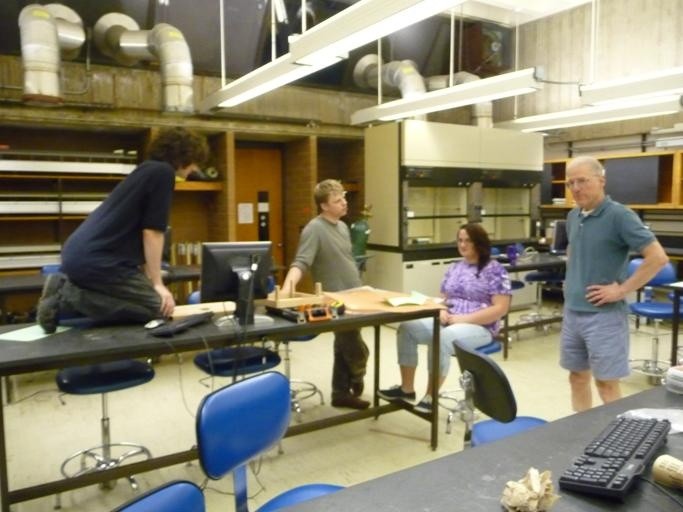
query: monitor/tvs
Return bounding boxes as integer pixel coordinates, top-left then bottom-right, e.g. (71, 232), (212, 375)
(550, 220), (568, 255)
(199, 241), (273, 327)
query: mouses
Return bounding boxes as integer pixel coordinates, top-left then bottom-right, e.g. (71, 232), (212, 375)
(144, 319), (165, 329)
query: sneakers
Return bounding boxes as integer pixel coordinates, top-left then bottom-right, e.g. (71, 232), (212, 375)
(353, 384), (363, 397)
(36, 272), (68, 334)
(414, 401), (433, 413)
(376, 387), (415, 404)
(331, 396), (370, 409)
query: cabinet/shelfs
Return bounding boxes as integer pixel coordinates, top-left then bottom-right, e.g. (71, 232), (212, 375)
(140, 124), (238, 363)
(542, 150), (683, 211)
(285, 135), (367, 295)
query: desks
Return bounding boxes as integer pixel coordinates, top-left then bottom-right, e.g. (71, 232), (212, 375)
(2, 286), (445, 511)
(281, 387), (681, 512)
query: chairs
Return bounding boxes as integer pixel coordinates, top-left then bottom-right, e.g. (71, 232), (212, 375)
(446, 340), (548, 450)
(492, 241), (566, 347)
(628, 259), (676, 376)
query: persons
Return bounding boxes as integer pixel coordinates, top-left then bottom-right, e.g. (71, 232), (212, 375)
(556, 156), (671, 411)
(35, 119), (211, 335)
(371, 222), (513, 415)
(276, 179), (375, 412)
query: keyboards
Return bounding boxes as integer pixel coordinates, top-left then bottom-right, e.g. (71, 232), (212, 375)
(558, 417), (671, 503)
(147, 312), (216, 336)
